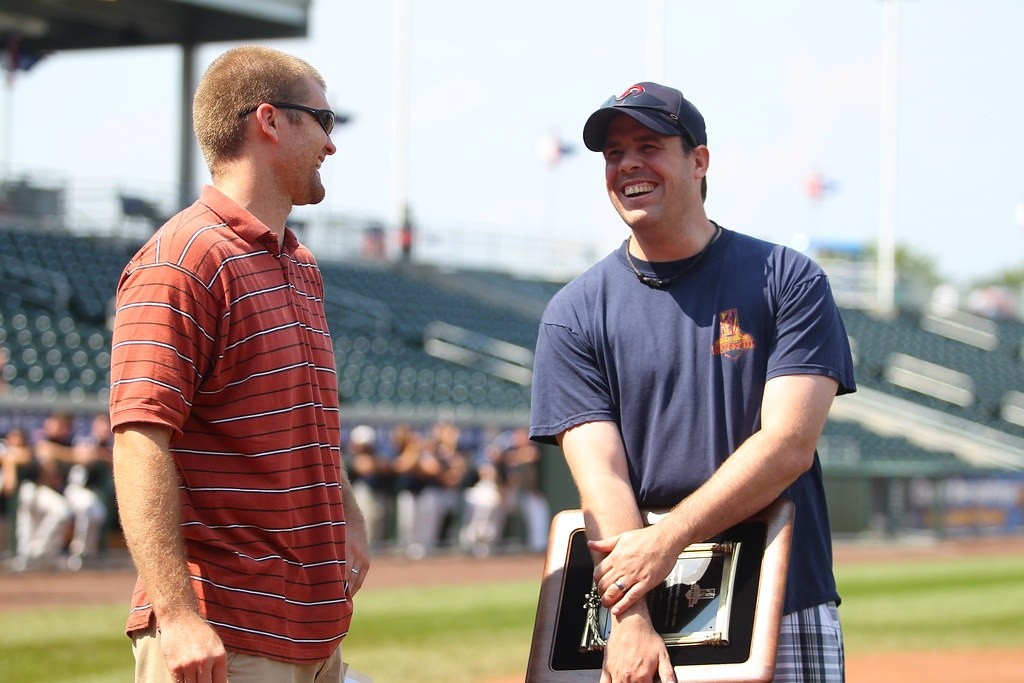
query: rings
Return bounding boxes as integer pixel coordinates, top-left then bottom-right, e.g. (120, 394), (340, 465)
(615, 579), (628, 595)
(350, 569), (360, 575)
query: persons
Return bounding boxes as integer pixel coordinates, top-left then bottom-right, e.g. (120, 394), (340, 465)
(108, 45), (374, 683)
(528, 82), (859, 683)
(0, 409), (554, 570)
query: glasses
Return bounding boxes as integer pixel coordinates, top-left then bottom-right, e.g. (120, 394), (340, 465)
(238, 101), (335, 137)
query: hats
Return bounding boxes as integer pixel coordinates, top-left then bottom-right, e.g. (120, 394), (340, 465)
(582, 81), (708, 152)
